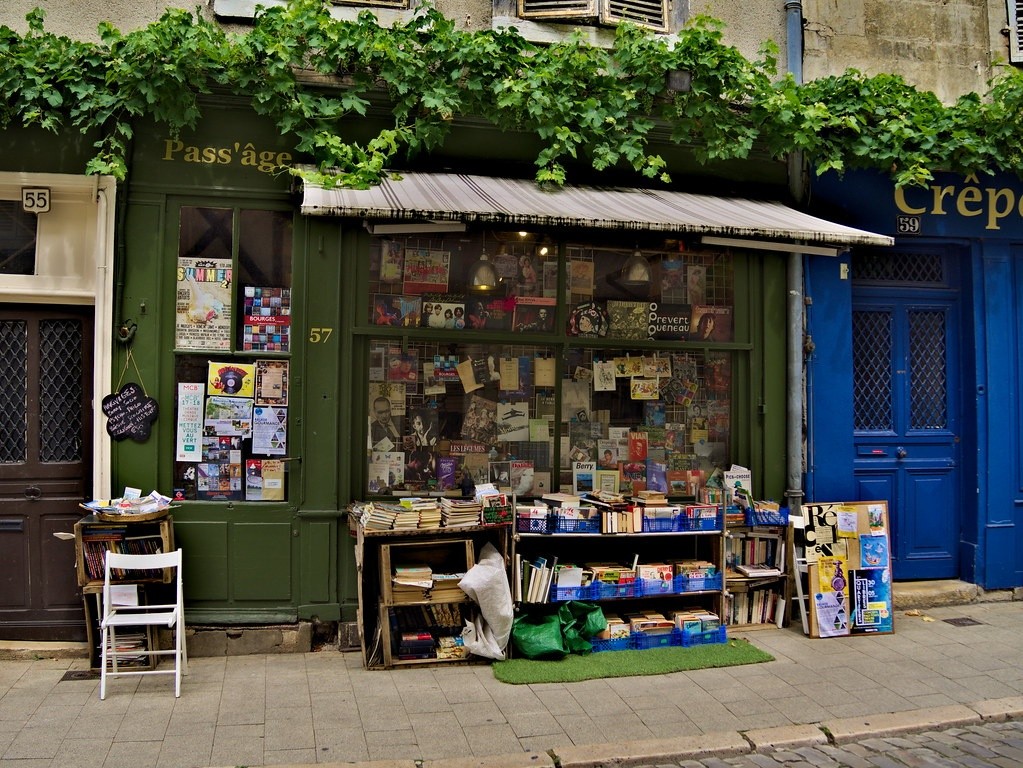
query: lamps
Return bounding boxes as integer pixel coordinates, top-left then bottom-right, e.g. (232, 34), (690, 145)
(468, 252), (499, 290)
(622, 245), (653, 285)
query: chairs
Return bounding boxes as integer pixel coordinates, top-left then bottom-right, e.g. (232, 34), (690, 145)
(100, 548), (187, 699)
(788, 514), (810, 633)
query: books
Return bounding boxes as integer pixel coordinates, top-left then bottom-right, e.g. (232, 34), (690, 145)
(362, 495), (482, 531)
(393, 567), (466, 602)
(515, 488), (787, 645)
(82, 524), (167, 666)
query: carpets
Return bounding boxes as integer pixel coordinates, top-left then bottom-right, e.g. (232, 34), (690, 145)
(492, 639), (774, 684)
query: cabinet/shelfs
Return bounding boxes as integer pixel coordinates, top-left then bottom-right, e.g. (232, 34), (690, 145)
(722, 522), (796, 633)
(505, 483), (728, 659)
(73, 514), (178, 674)
(355, 521), (509, 670)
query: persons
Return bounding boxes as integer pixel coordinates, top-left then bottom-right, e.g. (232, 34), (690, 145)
(599, 450), (617, 470)
(693, 406), (703, 418)
(423, 303), (464, 328)
(371, 397), (404, 441)
(690, 313), (716, 342)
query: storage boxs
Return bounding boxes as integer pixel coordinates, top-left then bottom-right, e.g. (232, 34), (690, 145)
(641, 509), (724, 533)
(744, 505), (791, 525)
(550, 571), (722, 602)
(512, 497), (602, 533)
(590, 625), (727, 651)
(398, 629), (467, 659)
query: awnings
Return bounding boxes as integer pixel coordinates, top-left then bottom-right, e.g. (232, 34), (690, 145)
(297, 163), (894, 250)
